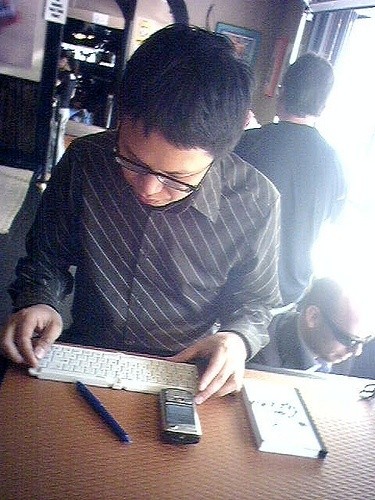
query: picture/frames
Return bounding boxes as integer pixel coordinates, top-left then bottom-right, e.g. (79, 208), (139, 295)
(214, 23), (262, 69)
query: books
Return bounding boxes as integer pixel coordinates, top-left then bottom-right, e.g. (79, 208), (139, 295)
(243, 377), (331, 459)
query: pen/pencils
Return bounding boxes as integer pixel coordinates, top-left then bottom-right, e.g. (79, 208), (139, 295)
(75, 380), (129, 444)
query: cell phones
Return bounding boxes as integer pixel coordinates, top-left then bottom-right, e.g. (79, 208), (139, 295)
(160, 387), (202, 446)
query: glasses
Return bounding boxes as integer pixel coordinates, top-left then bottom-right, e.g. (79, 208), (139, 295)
(361, 382), (375, 400)
(319, 309), (375, 352)
(112, 120), (217, 192)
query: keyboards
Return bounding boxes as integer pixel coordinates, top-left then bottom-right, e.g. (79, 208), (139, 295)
(26, 343), (201, 397)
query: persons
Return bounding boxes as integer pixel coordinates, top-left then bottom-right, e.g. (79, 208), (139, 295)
(0, 23), (284, 405)
(253, 273), (374, 379)
(46, 50), (79, 183)
(234, 51), (348, 313)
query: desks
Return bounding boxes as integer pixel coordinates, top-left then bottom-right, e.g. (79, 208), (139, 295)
(0, 338), (375, 500)
